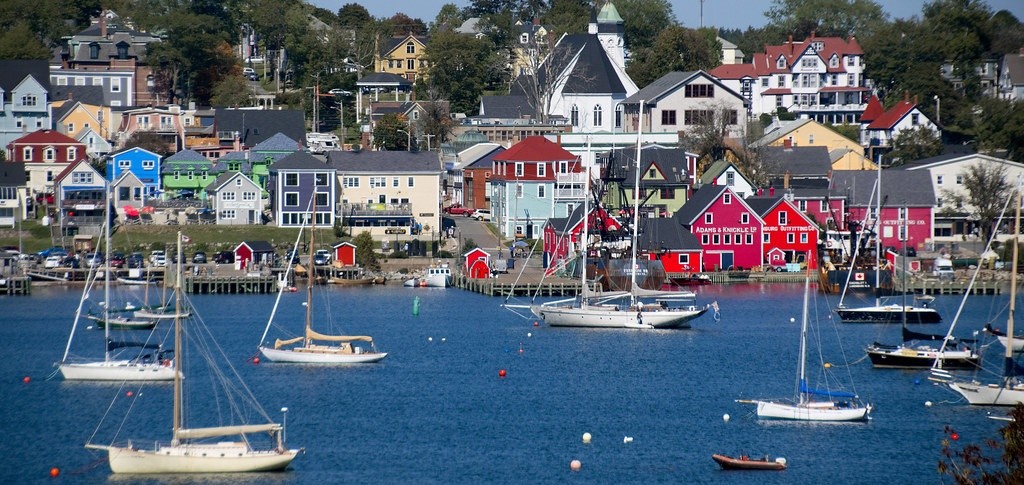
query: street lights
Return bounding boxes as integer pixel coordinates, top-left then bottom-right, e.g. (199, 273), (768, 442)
(398, 129), (410, 153)
(330, 107), (344, 150)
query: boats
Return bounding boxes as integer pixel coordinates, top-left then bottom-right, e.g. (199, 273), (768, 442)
(712, 455), (786, 472)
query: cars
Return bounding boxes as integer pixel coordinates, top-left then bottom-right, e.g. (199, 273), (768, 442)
(64, 256), (79, 269)
(154, 257), (164, 267)
(172, 251), (186, 264)
(126, 253), (143, 269)
(44, 257), (59, 269)
(242, 67), (258, 81)
(83, 252), (103, 268)
(109, 254), (125, 268)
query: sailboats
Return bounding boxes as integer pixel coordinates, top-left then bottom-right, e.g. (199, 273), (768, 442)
(834, 155), (941, 324)
(258, 170), (388, 364)
(85, 231), (304, 473)
(865, 197), (981, 371)
(500, 133), (708, 328)
(53, 181), (193, 380)
(757, 251), (872, 424)
(927, 179), (1024, 407)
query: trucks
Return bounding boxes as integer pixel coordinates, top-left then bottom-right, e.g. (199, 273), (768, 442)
(933, 258), (956, 281)
(762, 253), (807, 272)
(305, 132), (342, 154)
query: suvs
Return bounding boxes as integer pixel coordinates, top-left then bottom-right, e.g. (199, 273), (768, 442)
(471, 208), (491, 222)
(328, 89), (353, 97)
(191, 252), (208, 264)
(149, 251), (163, 262)
(217, 250), (235, 263)
(36, 193), (55, 205)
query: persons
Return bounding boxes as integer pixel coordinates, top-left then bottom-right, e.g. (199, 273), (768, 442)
(514, 247), (528, 258)
(510, 246), (514, 257)
(404, 243), (408, 255)
(419, 223), (423, 235)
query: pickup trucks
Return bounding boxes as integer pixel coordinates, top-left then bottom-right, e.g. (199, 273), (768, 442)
(443, 203), (473, 218)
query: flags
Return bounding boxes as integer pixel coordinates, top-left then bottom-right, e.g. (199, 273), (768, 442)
(182, 235), (192, 243)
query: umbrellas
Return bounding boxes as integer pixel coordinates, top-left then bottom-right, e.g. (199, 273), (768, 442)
(122, 205), (155, 217)
(513, 241), (528, 247)
